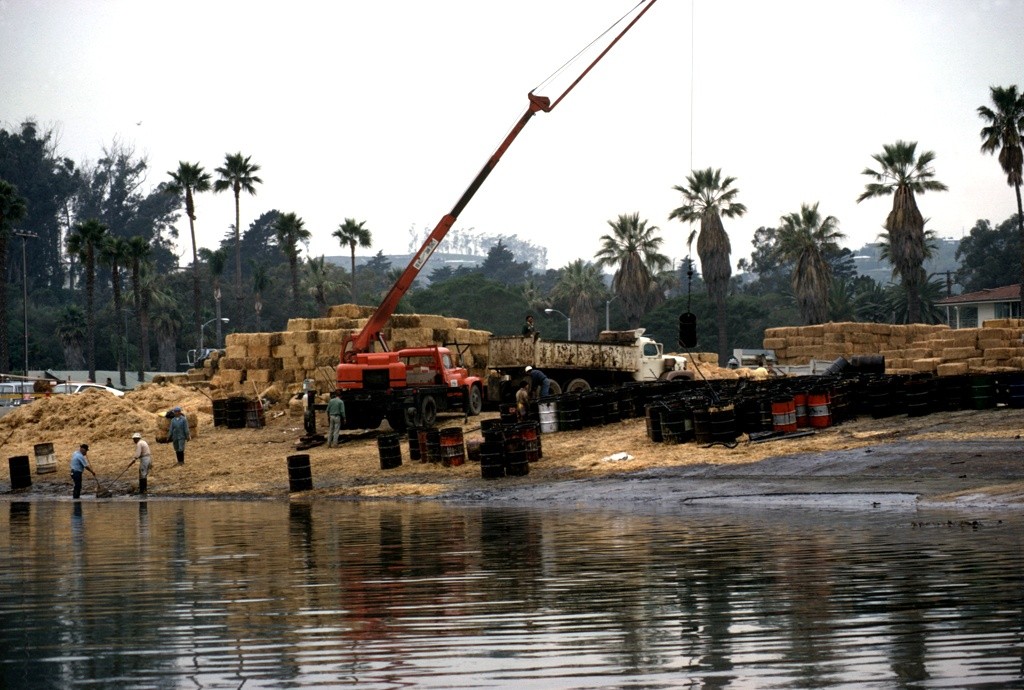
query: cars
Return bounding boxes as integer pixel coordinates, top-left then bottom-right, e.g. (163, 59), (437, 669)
(51, 382), (125, 397)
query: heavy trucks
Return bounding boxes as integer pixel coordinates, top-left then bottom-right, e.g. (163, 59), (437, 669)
(486, 327), (696, 410)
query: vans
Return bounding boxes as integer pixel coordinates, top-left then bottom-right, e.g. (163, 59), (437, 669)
(0, 382), (35, 409)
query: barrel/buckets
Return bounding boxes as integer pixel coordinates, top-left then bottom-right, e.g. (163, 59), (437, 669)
(213, 397), (266, 429)
(407, 426), (441, 464)
(287, 454), (313, 493)
(377, 433), (402, 469)
(680, 312), (697, 347)
(33, 443), (56, 474)
(439, 427), (465, 467)
(9, 502), (30, 527)
(8, 456), (32, 489)
(478, 356), (1024, 480)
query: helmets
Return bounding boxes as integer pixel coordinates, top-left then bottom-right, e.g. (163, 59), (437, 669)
(80, 444), (89, 450)
(525, 366), (533, 371)
(132, 433), (142, 438)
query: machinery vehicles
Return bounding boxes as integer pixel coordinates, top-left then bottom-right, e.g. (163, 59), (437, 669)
(293, 0), (656, 451)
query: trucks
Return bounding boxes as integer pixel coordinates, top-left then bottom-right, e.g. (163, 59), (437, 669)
(725, 346), (837, 375)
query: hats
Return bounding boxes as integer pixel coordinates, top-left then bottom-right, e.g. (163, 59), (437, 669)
(173, 408), (180, 412)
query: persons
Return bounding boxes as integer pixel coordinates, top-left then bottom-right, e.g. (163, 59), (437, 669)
(132, 432), (152, 494)
(487, 366), (550, 416)
(70, 444), (96, 499)
(753, 362), (778, 381)
(302, 390), (346, 448)
(44, 390), (51, 399)
(166, 407), (191, 466)
(106, 378), (114, 387)
(523, 315), (542, 368)
(810, 355), (817, 375)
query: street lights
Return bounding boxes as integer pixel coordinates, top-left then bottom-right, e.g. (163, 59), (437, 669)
(201, 317), (230, 348)
(544, 308), (571, 341)
